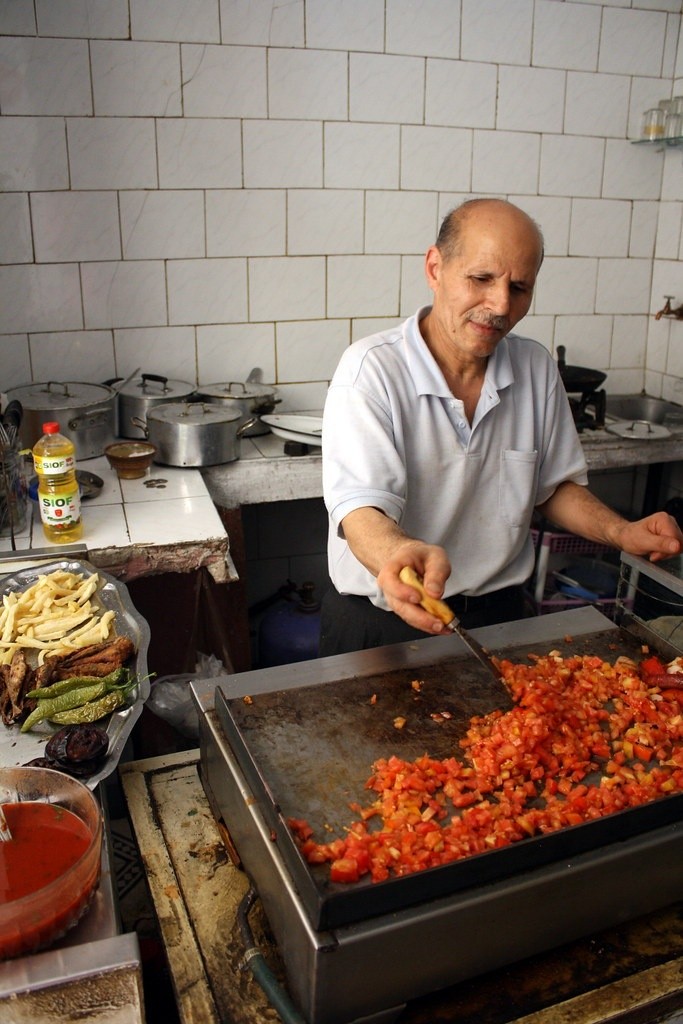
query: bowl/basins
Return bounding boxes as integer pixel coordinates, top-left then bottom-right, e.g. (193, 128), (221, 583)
(105, 441), (158, 479)
(1, 766), (103, 958)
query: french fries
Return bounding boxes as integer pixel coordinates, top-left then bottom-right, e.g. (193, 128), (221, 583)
(0, 568), (118, 666)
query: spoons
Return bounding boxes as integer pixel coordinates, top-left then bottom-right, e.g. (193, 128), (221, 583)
(2, 400), (24, 438)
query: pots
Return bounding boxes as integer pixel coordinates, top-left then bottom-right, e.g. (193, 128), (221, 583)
(196, 381), (278, 438)
(131, 401), (259, 468)
(2, 380), (119, 463)
(552, 345), (606, 392)
(112, 373), (197, 439)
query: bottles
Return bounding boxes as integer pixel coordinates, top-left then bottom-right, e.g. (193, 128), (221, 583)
(32, 422), (83, 542)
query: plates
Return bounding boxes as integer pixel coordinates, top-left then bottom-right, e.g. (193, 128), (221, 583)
(260, 415), (323, 447)
(30, 470), (104, 499)
(0, 558), (151, 806)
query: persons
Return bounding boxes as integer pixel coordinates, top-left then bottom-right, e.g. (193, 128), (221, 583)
(314, 198), (683, 659)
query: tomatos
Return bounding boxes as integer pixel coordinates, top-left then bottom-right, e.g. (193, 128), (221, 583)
(292, 651), (683, 883)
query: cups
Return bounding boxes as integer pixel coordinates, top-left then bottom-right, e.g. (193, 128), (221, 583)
(641, 96), (683, 139)
(0, 440), (26, 537)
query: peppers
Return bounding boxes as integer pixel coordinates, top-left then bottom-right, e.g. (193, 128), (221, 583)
(20, 668), (157, 733)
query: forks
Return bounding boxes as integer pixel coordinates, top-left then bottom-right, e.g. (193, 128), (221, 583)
(0, 423), (18, 526)
